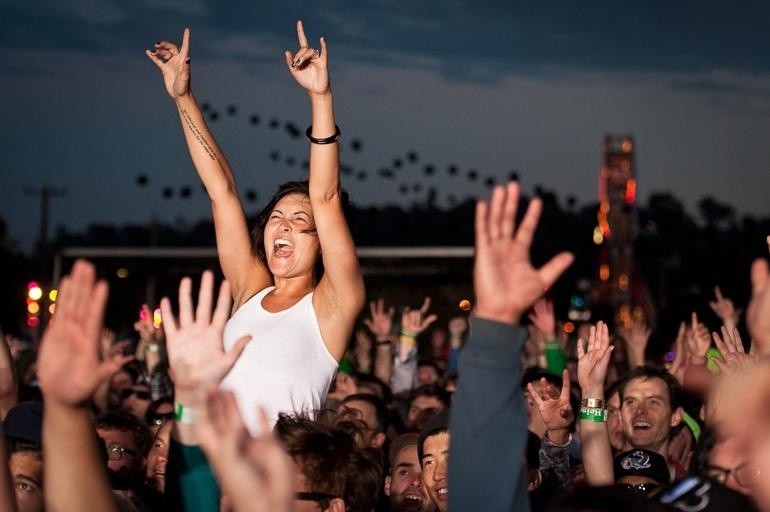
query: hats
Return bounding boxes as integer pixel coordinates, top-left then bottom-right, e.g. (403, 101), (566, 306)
(0, 401), (44, 444)
(389, 411), (451, 470)
(651, 475), (755, 511)
(614, 448), (671, 483)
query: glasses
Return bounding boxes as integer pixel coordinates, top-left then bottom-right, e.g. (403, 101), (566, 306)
(615, 483), (658, 498)
(121, 387), (152, 400)
(103, 443), (137, 462)
(147, 411), (177, 427)
(699, 462), (765, 489)
